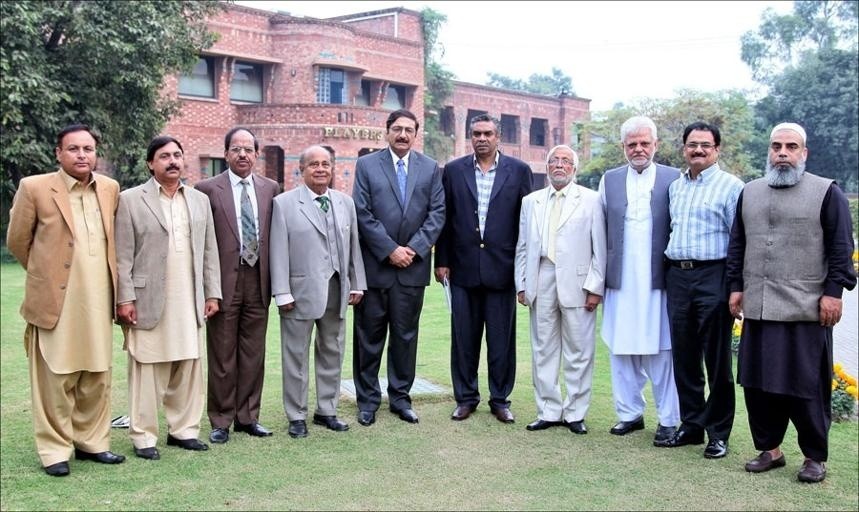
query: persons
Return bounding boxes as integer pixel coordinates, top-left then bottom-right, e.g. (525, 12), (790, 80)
(352, 112), (447, 427)
(5, 123), (124, 477)
(598, 115), (685, 445)
(725, 121), (855, 482)
(514, 145), (605, 434)
(116, 137), (223, 461)
(433, 113), (534, 424)
(194, 128), (279, 445)
(664, 122), (745, 458)
(268, 147), (367, 439)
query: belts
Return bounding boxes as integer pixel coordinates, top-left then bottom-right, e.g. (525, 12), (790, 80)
(669, 259), (726, 270)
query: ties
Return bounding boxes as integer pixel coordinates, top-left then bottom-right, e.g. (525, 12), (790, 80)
(397, 159), (407, 207)
(547, 191), (563, 265)
(316, 195), (329, 213)
(240, 179), (259, 268)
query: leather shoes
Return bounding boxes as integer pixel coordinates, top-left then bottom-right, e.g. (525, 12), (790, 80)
(357, 410), (376, 426)
(233, 422), (274, 437)
(562, 418), (588, 435)
(664, 426), (706, 448)
(43, 461), (70, 477)
(798, 458), (826, 482)
(134, 446), (160, 461)
(653, 424), (677, 448)
(744, 452), (787, 473)
(490, 406), (516, 423)
(74, 449), (126, 464)
(610, 415), (646, 436)
(390, 407), (418, 424)
(526, 419), (562, 431)
(288, 421), (309, 439)
(209, 428), (230, 445)
(312, 412), (350, 432)
(166, 433), (210, 452)
(703, 438), (729, 459)
(452, 405), (477, 420)
